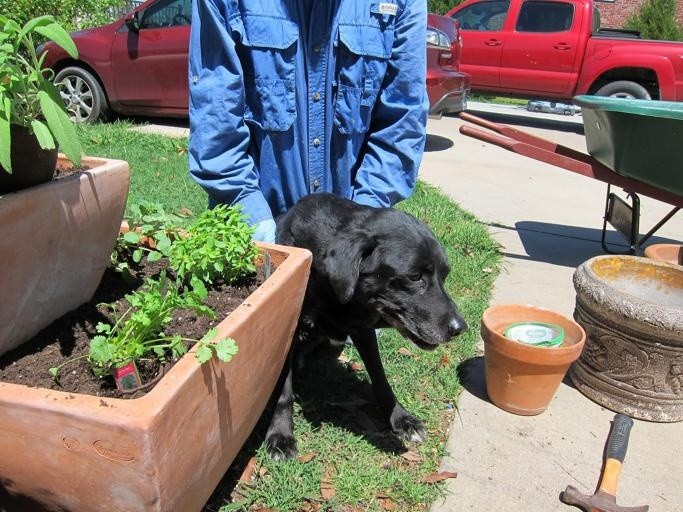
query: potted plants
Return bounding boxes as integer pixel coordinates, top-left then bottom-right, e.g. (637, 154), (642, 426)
(1, 200), (312, 512)
(2, 14), (130, 352)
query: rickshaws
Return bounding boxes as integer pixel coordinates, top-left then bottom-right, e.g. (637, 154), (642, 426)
(458, 96), (683, 256)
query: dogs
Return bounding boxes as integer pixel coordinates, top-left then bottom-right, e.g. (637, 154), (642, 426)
(265, 187), (469, 463)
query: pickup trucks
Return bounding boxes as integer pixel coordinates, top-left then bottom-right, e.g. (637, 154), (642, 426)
(431, 0), (683, 109)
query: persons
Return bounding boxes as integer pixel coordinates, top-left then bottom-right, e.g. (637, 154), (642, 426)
(188, 0), (430, 384)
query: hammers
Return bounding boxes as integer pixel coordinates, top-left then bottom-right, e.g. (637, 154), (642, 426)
(562, 414), (649, 512)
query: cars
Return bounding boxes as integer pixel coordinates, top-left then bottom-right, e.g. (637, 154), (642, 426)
(27, 0), (472, 128)
(528, 100), (575, 115)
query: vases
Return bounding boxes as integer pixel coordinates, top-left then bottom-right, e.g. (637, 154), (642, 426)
(646, 243), (682, 271)
(480, 304), (586, 417)
(573, 254), (683, 422)
